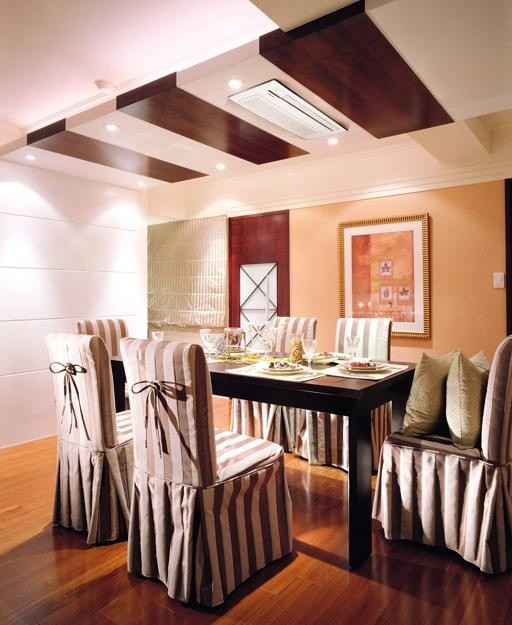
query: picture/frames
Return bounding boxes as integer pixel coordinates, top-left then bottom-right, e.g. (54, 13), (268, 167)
(338, 212), (431, 339)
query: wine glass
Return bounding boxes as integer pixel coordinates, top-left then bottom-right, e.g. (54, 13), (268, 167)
(199, 329), (211, 354)
(205, 334), (216, 364)
(302, 339), (316, 372)
(346, 336), (360, 362)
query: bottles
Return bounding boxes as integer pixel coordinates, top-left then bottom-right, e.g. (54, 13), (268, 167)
(216, 334), (225, 357)
(290, 334), (304, 364)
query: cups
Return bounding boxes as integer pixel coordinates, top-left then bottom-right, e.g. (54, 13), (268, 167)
(152, 331), (164, 341)
(224, 327), (246, 353)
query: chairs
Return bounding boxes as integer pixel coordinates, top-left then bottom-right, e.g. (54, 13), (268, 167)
(119, 337), (294, 609)
(231, 316), (317, 451)
(372, 334), (512, 573)
(301, 316), (393, 475)
(78, 318), (127, 359)
(45, 335), (133, 546)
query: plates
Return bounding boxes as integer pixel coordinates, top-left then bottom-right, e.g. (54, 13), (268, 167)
(261, 353), (391, 375)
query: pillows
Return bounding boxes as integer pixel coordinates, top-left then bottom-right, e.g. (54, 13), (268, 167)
(445, 351), (489, 449)
(403, 349), (458, 437)
